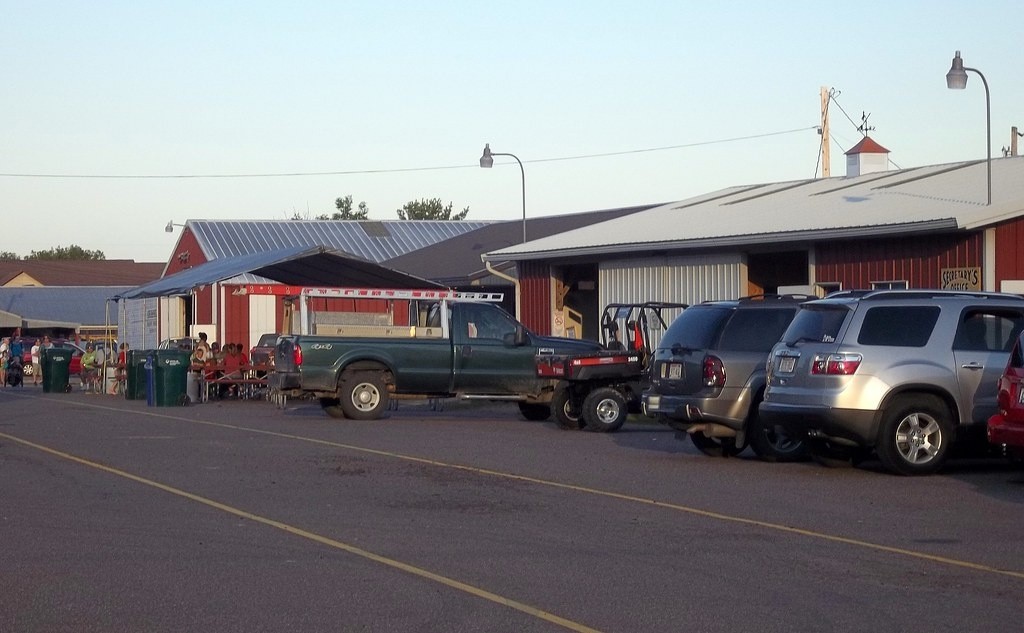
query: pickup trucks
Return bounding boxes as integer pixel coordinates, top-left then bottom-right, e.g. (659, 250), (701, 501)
(250, 296), (613, 421)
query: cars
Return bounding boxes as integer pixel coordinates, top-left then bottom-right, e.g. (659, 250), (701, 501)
(158, 337), (199, 350)
(71, 337), (118, 357)
(0, 337), (85, 376)
(985, 328), (1024, 468)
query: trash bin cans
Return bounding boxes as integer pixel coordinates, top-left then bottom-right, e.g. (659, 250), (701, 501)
(124, 350), (153, 400)
(41, 348), (76, 393)
(153, 349), (195, 407)
(145, 356), (153, 406)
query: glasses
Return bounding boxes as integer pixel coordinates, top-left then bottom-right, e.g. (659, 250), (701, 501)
(87, 347), (93, 349)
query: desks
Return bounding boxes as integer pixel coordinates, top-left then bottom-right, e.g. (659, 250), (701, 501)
(205, 366), (273, 400)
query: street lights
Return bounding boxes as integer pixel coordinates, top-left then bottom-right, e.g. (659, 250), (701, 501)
(945, 50), (993, 205)
(479, 144), (528, 246)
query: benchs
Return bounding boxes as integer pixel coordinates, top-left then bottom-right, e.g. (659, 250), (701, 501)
(202, 379), (273, 403)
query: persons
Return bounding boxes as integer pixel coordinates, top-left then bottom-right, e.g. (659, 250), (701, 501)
(187, 332), (250, 400)
(95, 341), (118, 364)
(0, 335), (23, 386)
(30, 338), (43, 385)
(38, 335), (56, 359)
(118, 343), (130, 364)
(79, 344), (97, 385)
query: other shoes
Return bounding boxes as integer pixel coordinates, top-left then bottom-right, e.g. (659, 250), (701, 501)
(34, 381), (37, 385)
(80, 384), (87, 391)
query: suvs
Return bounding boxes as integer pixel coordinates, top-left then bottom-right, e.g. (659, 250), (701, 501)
(639, 292), (826, 460)
(757, 286), (1024, 476)
